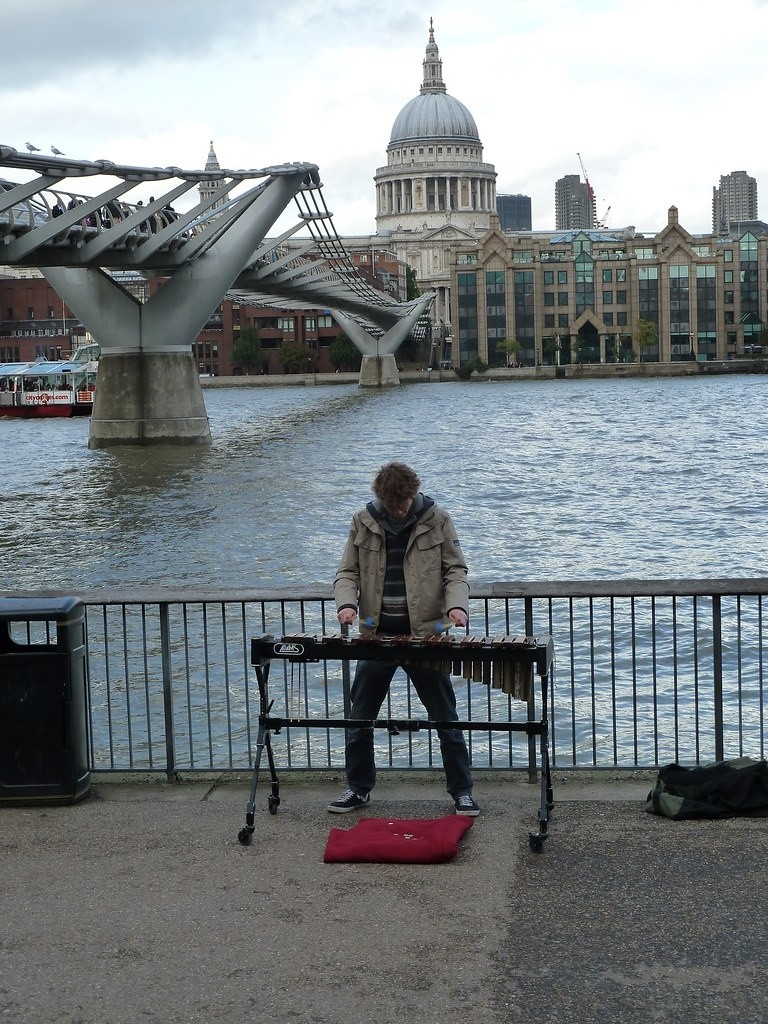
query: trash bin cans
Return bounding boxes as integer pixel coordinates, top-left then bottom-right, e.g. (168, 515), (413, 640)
(556, 367), (565, 378)
(0, 596), (92, 807)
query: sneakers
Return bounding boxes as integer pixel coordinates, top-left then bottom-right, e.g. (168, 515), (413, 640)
(327, 788), (371, 812)
(454, 795), (480, 816)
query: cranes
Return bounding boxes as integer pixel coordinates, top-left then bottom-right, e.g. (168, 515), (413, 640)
(577, 152), (611, 228)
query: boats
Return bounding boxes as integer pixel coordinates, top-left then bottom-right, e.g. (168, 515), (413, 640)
(0, 360), (99, 417)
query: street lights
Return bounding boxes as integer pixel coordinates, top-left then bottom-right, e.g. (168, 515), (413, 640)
(308, 357), (312, 374)
(536, 349), (540, 366)
(689, 332), (695, 361)
(432, 342), (438, 370)
(751, 342), (754, 360)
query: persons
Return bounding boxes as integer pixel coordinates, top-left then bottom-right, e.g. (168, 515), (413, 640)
(327, 462), (482, 816)
(444, 362), (458, 370)
(0, 378), (87, 392)
(52, 196), (200, 239)
(508, 361), (523, 368)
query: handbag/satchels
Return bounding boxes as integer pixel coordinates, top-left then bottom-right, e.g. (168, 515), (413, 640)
(643, 757), (768, 820)
